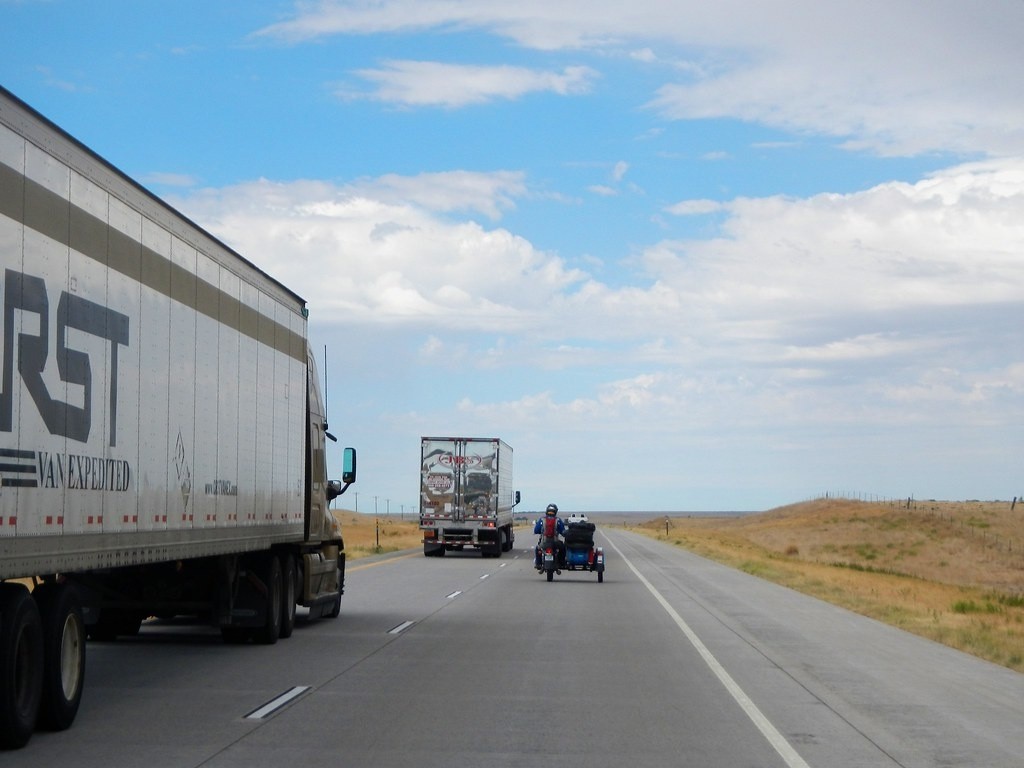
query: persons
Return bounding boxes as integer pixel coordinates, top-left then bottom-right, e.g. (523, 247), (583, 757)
(531, 504), (566, 574)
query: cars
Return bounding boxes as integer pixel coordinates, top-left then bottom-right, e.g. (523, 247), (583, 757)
(572, 513), (585, 517)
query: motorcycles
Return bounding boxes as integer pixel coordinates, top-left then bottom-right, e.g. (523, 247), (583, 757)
(532, 518), (605, 583)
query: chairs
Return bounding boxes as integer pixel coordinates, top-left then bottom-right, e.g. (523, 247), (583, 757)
(563, 522), (595, 550)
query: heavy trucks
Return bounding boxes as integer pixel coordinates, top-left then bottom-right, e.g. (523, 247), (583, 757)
(420, 436), (522, 558)
(0, 87), (356, 753)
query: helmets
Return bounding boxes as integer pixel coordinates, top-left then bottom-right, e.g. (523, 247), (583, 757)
(546, 503), (558, 516)
